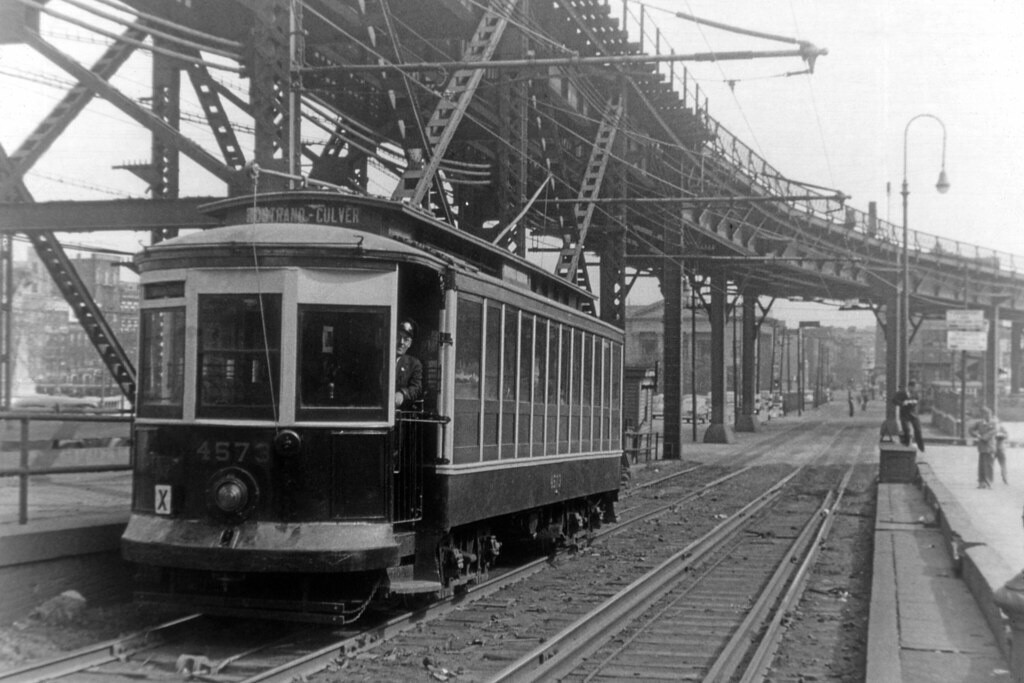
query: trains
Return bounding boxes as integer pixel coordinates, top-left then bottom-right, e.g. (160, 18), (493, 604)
(119, 194), (626, 626)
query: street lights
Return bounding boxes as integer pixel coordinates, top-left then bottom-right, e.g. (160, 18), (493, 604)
(898, 113), (949, 437)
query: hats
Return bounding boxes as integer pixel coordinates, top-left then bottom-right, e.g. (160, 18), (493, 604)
(397, 316), (419, 339)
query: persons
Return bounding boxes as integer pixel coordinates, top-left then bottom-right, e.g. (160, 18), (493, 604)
(395, 315), (423, 409)
(969, 405), (1007, 489)
(892, 378), (924, 452)
